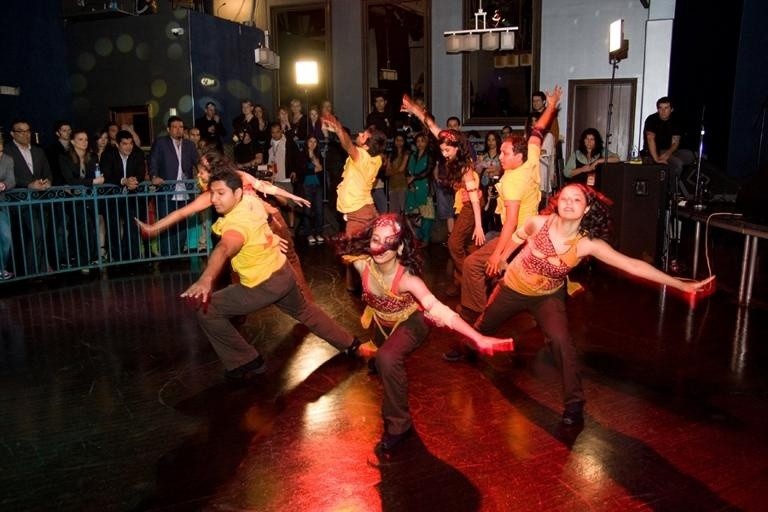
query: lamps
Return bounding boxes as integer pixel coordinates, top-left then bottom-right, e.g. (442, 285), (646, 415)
(254, 31), (280, 71)
(604, 17), (629, 169)
(379, 34), (399, 81)
(444, 1), (519, 53)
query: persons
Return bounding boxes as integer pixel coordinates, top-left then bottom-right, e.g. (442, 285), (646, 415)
(444, 86), (716, 445)
(2, 87), (476, 283)
(135, 152), (361, 374)
(341, 213), (514, 449)
(400, 97), (485, 296)
(323, 113), (386, 291)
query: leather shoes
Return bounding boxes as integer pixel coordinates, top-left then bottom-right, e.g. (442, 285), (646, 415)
(308, 233), (325, 242)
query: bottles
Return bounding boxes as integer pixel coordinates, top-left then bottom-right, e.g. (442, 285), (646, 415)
(94, 162), (102, 179)
(631, 145), (641, 160)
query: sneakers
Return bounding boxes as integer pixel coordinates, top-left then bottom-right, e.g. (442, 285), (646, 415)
(1, 270), (12, 280)
(344, 334), (368, 362)
(558, 399), (586, 427)
(366, 345), (383, 376)
(224, 353), (270, 379)
(373, 422), (417, 458)
(440, 343), (470, 362)
